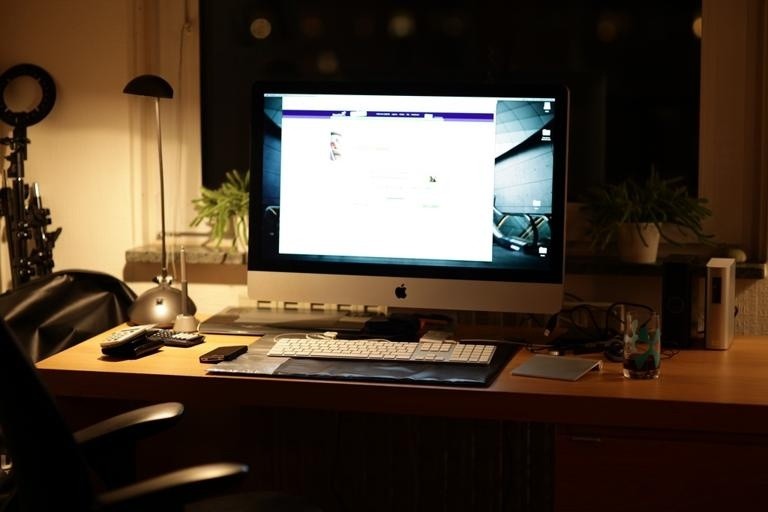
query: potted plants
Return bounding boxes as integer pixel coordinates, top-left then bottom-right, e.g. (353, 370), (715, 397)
(191, 167), (250, 252)
(582, 172), (716, 263)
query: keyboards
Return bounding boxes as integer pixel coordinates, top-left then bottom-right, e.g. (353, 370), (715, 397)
(267, 338), (497, 365)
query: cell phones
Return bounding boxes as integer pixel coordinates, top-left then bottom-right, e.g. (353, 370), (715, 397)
(199, 346), (248, 363)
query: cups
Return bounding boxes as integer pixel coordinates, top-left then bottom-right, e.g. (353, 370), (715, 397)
(623, 311), (663, 379)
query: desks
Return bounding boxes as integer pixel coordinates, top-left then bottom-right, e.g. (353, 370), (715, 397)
(33, 320), (768, 512)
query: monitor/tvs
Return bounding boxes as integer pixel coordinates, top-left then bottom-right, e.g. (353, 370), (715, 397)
(247, 81), (570, 342)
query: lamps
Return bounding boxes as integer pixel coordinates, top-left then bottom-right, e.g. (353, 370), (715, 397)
(122, 74), (196, 328)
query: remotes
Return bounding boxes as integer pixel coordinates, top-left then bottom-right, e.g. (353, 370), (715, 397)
(99, 324), (159, 348)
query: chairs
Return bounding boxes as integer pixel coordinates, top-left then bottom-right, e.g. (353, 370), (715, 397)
(0, 319), (325, 512)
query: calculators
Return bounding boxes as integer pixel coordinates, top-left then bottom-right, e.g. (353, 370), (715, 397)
(100, 326), (146, 347)
